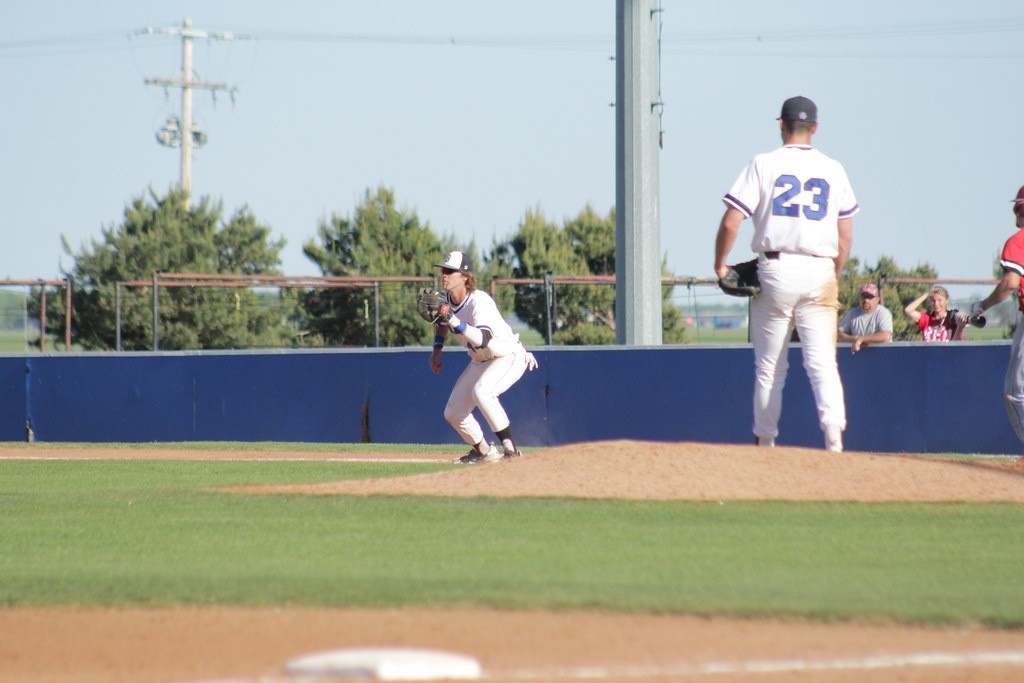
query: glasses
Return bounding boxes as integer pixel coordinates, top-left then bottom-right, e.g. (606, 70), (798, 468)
(861, 293), (877, 299)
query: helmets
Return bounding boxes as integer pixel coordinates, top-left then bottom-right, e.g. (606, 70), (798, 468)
(1010, 185), (1024, 217)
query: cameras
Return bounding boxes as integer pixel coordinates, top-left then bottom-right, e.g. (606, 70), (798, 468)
(949, 309), (986, 329)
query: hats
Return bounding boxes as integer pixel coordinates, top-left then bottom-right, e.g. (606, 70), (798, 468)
(434, 251), (473, 271)
(776, 96), (817, 123)
(860, 283), (877, 295)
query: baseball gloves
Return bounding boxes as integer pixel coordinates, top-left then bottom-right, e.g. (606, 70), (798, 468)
(415, 285), (455, 328)
(716, 255), (762, 297)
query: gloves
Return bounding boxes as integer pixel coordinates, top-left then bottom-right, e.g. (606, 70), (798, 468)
(970, 301), (984, 320)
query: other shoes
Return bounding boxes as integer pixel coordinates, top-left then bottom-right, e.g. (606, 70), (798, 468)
(756, 437), (774, 447)
(824, 424), (843, 451)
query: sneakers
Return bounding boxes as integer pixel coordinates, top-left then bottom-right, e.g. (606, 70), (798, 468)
(500, 450), (522, 460)
(452, 444), (500, 464)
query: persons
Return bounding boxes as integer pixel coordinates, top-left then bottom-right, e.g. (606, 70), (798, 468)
(715, 97), (860, 454)
(422, 251), (532, 467)
(838, 282), (893, 353)
(967, 186), (1024, 447)
(904, 285), (966, 343)
